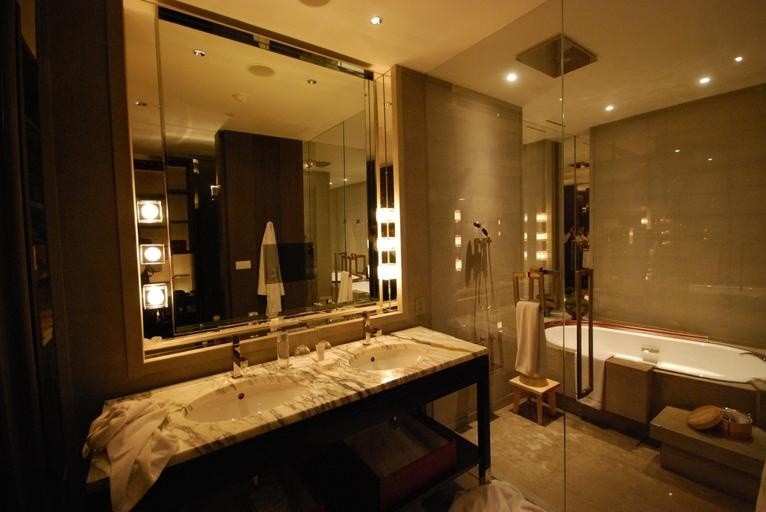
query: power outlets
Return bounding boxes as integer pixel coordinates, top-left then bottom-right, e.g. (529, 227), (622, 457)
(416, 297), (425, 315)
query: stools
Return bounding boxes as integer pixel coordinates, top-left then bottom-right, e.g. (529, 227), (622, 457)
(508, 373), (561, 425)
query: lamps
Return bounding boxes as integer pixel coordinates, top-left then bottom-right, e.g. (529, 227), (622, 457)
(137, 199), (169, 310)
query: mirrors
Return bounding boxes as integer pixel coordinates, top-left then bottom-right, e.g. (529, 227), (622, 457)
(105, 0), (410, 380)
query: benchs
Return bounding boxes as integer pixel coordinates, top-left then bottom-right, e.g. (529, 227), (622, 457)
(648, 405), (766, 504)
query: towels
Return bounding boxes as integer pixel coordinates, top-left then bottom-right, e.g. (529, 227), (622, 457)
(513, 300), (547, 379)
(336, 270), (354, 304)
(81, 400), (179, 512)
(574, 348), (614, 411)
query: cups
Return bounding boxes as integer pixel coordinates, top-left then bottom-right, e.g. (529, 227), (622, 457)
(315, 343), (325, 361)
(239, 360), (248, 378)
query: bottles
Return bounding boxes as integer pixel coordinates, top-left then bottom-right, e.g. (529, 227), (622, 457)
(362, 312), (372, 346)
(232, 335), (242, 378)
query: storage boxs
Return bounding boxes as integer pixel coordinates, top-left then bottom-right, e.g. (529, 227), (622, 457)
(687, 405), (752, 440)
